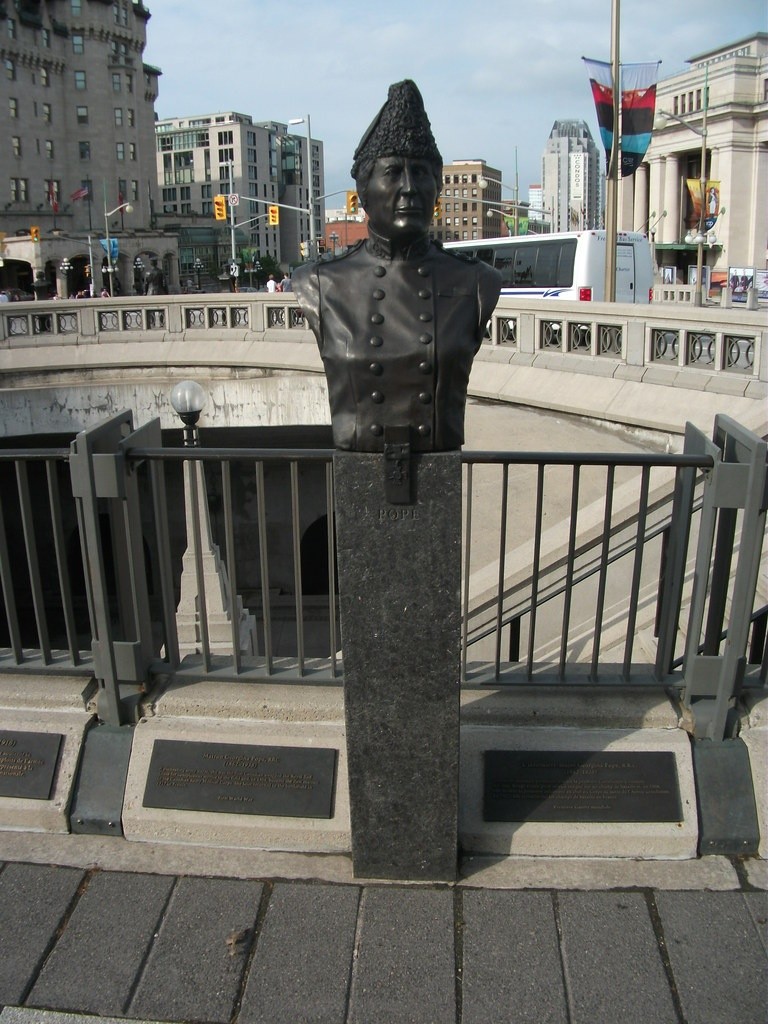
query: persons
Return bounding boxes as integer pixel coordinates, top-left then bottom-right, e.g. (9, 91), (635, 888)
(296, 82), (500, 452)
(266, 273), (278, 292)
(280, 274), (293, 291)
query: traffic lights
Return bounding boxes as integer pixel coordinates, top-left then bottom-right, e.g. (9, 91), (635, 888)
(269, 206), (280, 226)
(346, 191), (360, 216)
(432, 199), (444, 220)
(214, 196), (226, 220)
(31, 225), (41, 244)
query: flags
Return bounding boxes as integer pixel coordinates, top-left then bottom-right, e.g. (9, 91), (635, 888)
(47, 183), (57, 213)
(71, 186), (88, 200)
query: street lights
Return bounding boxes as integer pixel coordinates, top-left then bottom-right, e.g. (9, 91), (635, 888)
(253, 262), (264, 291)
(288, 114), (323, 263)
(193, 258), (204, 289)
(478, 144), (519, 237)
(134, 258), (146, 295)
(105, 202), (133, 296)
(60, 257), (74, 299)
(170, 380), (214, 675)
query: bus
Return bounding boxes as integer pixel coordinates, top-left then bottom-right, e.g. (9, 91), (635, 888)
(442, 229), (655, 347)
